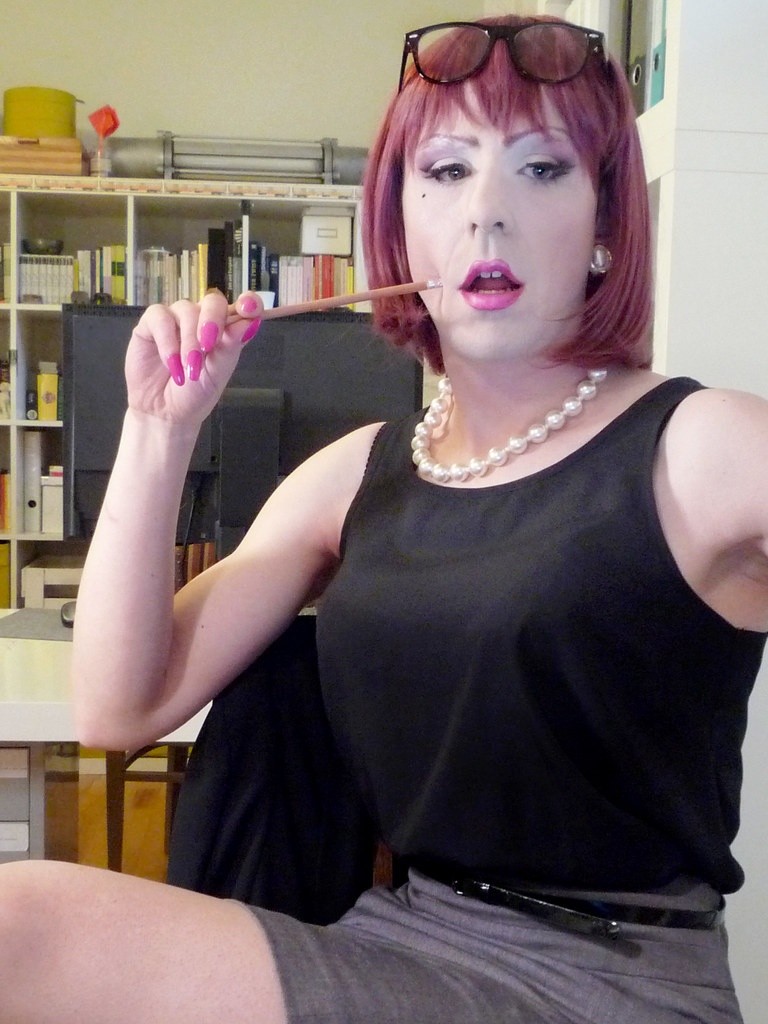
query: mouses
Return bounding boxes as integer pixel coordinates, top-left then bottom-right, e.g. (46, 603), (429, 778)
(61, 601), (77, 627)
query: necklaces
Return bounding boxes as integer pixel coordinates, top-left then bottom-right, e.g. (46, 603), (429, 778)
(410, 363), (609, 484)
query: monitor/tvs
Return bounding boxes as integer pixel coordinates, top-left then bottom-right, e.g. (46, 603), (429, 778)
(60, 303), (423, 564)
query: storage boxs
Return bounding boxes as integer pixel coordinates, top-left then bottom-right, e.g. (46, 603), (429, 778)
(21, 553), (86, 607)
(39, 476), (65, 537)
(0, 135), (90, 176)
(298, 205), (354, 256)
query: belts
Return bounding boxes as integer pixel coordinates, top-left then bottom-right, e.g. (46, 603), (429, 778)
(414, 865), (727, 943)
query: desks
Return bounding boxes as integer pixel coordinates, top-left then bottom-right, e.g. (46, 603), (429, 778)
(0, 608), (215, 885)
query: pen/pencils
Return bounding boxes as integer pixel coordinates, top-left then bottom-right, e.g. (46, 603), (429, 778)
(226, 278), (443, 327)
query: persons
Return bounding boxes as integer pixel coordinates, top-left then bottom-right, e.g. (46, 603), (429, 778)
(0, 14), (768, 1024)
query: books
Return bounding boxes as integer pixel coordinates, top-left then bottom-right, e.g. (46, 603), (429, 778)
(0, 218), (353, 595)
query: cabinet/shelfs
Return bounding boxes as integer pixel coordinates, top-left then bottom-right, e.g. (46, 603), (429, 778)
(533, 0), (768, 395)
(0, 176), (445, 606)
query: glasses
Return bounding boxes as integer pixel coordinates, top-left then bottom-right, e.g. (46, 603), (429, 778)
(398, 21), (609, 93)
